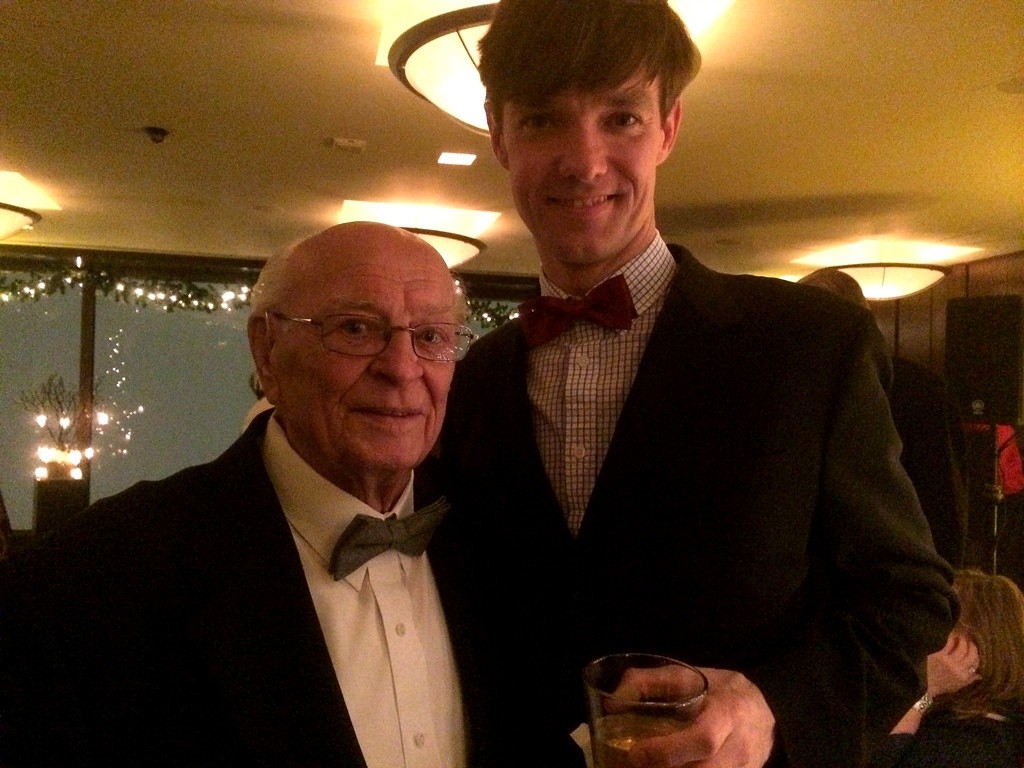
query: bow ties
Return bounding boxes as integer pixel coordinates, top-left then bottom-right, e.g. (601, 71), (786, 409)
(518, 274), (641, 351)
(329, 496), (454, 583)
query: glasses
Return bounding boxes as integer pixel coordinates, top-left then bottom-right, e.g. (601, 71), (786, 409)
(264, 309), (478, 364)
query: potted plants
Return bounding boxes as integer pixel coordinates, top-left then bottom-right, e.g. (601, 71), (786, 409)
(9, 372), (118, 537)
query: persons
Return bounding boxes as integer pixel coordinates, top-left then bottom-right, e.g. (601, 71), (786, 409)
(2, 221), (594, 767)
(785, 263), (966, 610)
(406, 0), (962, 768)
(976, 411), (1022, 576)
(872, 568), (1024, 768)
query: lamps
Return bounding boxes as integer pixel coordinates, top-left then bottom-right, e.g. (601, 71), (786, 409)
(397, 226), (488, 271)
(816, 262), (950, 304)
(0, 200), (44, 243)
(384, 2), (498, 138)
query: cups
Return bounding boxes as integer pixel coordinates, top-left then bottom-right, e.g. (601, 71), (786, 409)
(584, 652), (709, 768)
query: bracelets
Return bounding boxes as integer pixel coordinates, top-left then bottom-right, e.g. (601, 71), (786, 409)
(907, 684), (934, 713)
